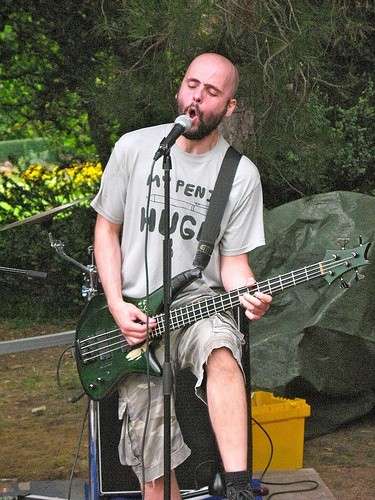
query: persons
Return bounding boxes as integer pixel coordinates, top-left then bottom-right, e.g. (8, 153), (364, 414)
(90, 52), (271, 500)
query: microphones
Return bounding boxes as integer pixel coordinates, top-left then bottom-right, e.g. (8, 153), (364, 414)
(153, 114), (192, 162)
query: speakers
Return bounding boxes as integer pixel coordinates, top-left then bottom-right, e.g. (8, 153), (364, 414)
(95, 306), (252, 496)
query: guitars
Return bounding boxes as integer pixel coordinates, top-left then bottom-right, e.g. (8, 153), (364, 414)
(76, 235), (372, 402)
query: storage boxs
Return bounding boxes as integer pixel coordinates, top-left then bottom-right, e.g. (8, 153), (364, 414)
(251, 391), (311, 473)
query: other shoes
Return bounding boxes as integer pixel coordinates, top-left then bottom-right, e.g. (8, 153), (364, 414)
(227, 483), (255, 500)
